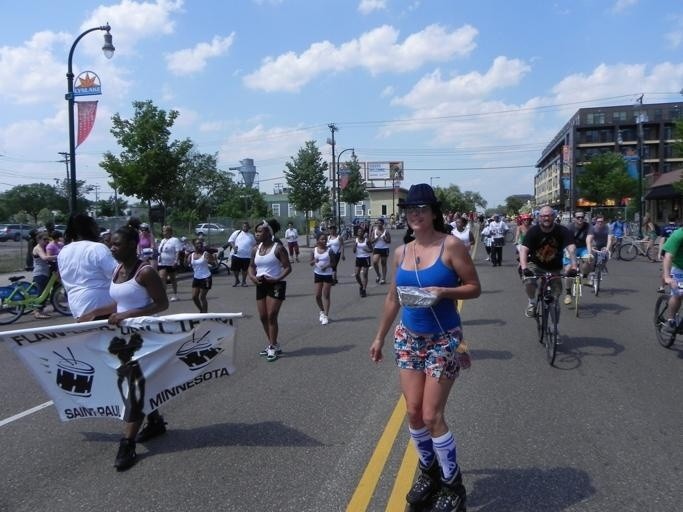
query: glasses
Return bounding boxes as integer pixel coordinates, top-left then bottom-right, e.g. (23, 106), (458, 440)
(406, 205), (430, 214)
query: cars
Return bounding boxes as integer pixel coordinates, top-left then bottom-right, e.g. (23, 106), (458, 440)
(194, 221), (235, 238)
(0, 220), (69, 246)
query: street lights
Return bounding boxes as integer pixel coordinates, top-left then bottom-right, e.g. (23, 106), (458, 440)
(335, 146), (357, 238)
(428, 175), (442, 188)
(65, 23), (114, 219)
(390, 171), (400, 215)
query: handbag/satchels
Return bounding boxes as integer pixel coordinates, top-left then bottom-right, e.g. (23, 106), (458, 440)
(453, 339), (473, 371)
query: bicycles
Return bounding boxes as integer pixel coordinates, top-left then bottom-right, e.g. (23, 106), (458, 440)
(519, 269), (573, 366)
(0, 261), (70, 327)
(653, 286), (683, 349)
(565, 226), (661, 318)
(501, 229), (513, 246)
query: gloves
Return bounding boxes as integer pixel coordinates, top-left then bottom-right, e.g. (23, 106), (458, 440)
(567, 269), (577, 277)
(524, 268), (534, 277)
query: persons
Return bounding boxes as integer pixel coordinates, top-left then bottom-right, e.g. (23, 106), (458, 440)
(226, 221), (302, 361)
(440, 209), (475, 252)
(136, 224), (182, 301)
(188, 241), (216, 313)
(642, 211), (683, 329)
(478, 212), (509, 267)
(352, 219), (391, 297)
(310, 225), (344, 324)
(31, 214), (112, 324)
(403, 224), (417, 244)
(370, 183), (480, 512)
(110, 218), (166, 472)
(513, 207), (627, 344)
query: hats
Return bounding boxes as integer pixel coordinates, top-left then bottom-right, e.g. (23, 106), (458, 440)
(521, 214), (532, 219)
(397, 184), (436, 208)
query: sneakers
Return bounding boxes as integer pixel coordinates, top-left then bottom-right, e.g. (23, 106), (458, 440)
(430, 469), (466, 512)
(405, 460), (440, 506)
(232, 276), (386, 362)
(525, 303), (537, 316)
(564, 295), (572, 305)
(114, 438), (137, 467)
(667, 320), (676, 330)
(137, 416), (167, 443)
(552, 332), (562, 345)
